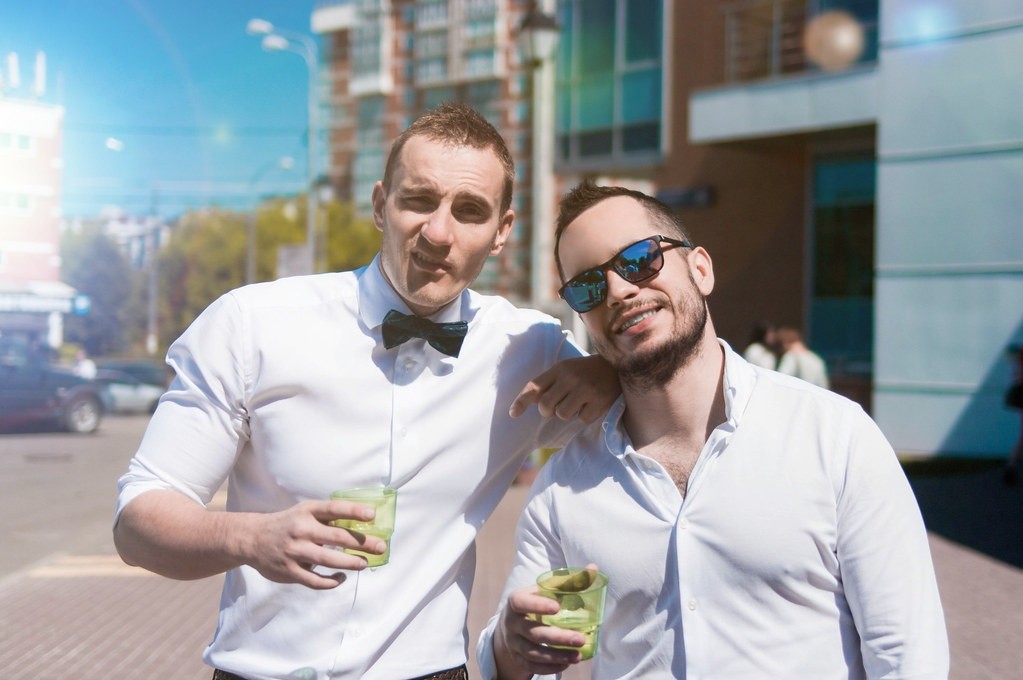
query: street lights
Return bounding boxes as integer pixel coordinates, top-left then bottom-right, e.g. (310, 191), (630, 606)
(245, 17), (316, 280)
(107, 139), (158, 355)
(508, 2), (565, 302)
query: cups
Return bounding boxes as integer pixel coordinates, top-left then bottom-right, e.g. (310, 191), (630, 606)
(328, 487), (398, 567)
(534, 568), (608, 661)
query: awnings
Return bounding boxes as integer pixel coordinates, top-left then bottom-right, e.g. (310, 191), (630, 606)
(0, 312), (50, 335)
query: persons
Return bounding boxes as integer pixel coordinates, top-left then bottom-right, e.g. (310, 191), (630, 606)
(29, 336), (47, 369)
(72, 347), (98, 381)
(475, 177), (950, 680)
(743, 326), (828, 388)
(108, 96), (626, 680)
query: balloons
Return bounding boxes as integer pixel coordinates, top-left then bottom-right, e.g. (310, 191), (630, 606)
(803, 11), (862, 71)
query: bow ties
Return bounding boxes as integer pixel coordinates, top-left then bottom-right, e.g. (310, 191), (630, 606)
(381, 309), (468, 358)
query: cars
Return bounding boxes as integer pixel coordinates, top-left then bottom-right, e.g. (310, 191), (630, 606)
(89, 359), (173, 414)
(0, 338), (114, 435)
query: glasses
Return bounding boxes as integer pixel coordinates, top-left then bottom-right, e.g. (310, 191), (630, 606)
(557, 235), (690, 313)
